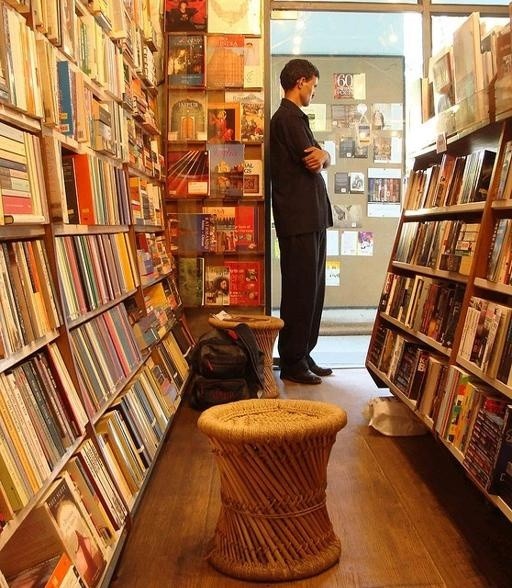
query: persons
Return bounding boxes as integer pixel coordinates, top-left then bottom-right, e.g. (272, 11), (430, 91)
(272, 57), (335, 386)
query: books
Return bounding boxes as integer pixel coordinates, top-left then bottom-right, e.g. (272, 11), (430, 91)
(408, 12), (512, 122)
(367, 140), (512, 503)
(1, 1), (264, 588)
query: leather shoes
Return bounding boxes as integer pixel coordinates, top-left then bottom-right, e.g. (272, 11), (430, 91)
(280, 367), (322, 384)
(310, 362), (332, 376)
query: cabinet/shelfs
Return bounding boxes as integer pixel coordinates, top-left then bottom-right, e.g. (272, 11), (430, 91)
(362, 74), (512, 559)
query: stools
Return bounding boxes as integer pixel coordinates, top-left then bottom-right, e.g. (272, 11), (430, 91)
(195, 396), (348, 581)
(209, 314), (285, 398)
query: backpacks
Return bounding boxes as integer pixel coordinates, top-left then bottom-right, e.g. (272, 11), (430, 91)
(187, 324), (264, 410)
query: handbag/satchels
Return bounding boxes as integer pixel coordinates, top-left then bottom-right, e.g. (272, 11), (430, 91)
(368, 397), (427, 436)
